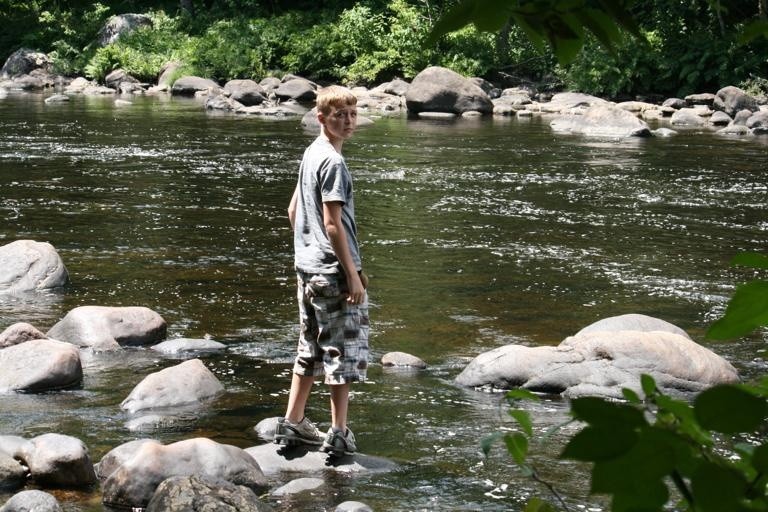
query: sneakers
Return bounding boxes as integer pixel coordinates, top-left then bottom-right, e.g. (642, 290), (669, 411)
(272, 416), (326, 446)
(319, 425), (356, 458)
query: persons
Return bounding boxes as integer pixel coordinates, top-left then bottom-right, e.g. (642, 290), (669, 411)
(274, 84), (370, 459)
